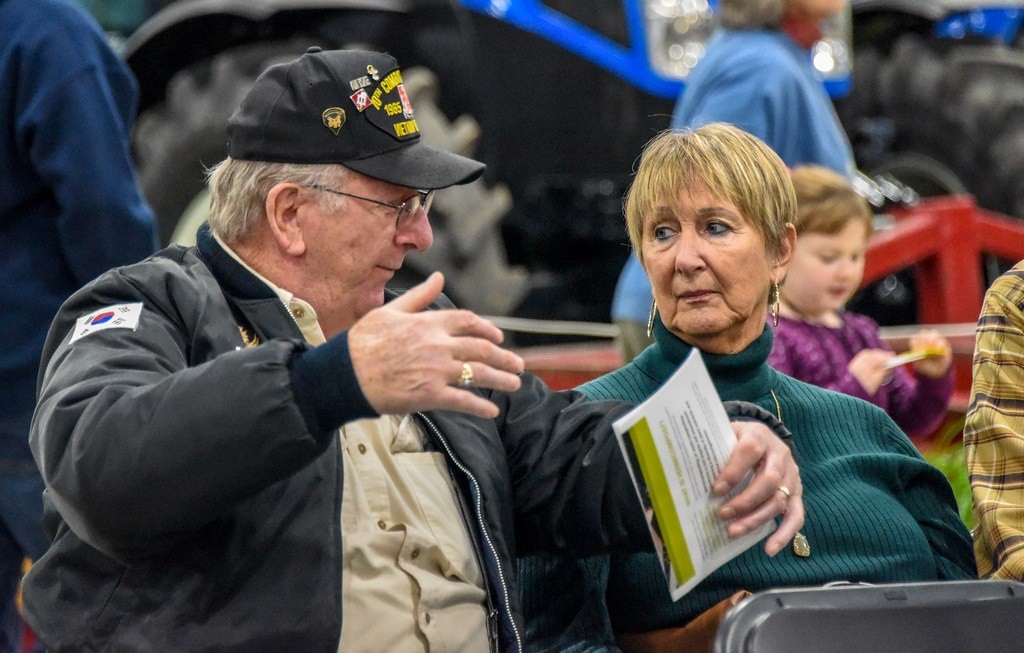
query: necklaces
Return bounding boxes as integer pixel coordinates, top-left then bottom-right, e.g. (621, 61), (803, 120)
(770, 389), (811, 557)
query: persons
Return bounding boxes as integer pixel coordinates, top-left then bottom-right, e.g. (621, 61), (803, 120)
(0, 0), (1024, 653)
(517, 122), (980, 653)
(20, 47), (806, 653)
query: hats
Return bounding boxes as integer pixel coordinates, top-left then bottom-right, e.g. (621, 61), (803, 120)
(225, 46), (486, 189)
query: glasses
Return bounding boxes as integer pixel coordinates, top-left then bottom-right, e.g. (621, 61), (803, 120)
(313, 185), (435, 231)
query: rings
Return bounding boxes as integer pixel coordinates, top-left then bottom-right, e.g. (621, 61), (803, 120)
(457, 362), (474, 387)
(778, 484), (791, 496)
